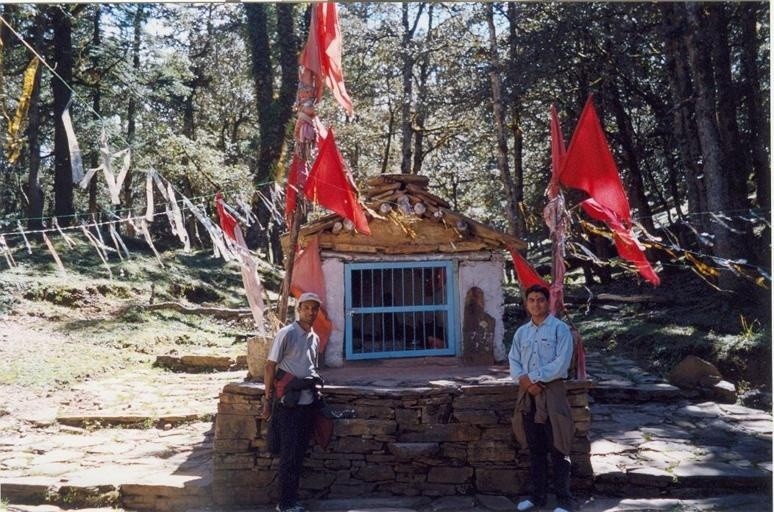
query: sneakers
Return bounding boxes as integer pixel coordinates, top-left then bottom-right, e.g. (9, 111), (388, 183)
(517, 499), (570, 512)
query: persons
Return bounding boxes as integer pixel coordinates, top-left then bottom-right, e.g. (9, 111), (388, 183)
(261, 290), (323, 511)
(507, 283), (578, 510)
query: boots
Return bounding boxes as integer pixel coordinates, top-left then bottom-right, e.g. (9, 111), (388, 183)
(275, 500), (310, 512)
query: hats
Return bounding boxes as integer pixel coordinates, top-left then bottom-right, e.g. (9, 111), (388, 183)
(298, 292), (321, 306)
